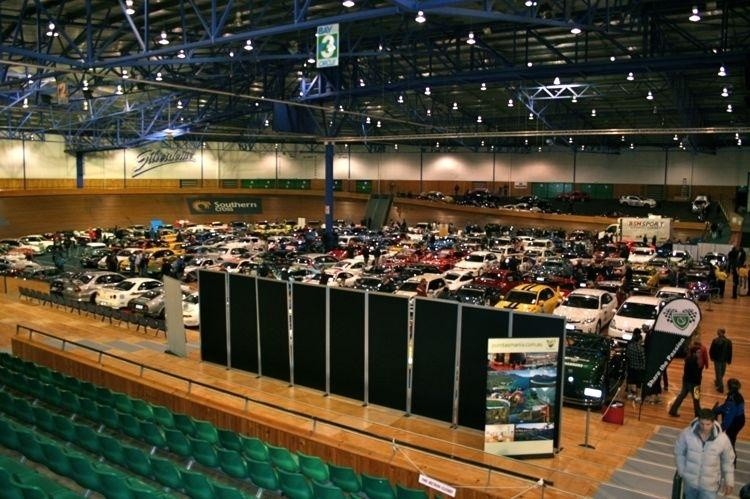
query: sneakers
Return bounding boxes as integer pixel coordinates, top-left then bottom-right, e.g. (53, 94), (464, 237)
(624, 388), (665, 405)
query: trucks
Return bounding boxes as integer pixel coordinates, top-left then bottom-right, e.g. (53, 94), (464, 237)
(599, 217), (671, 245)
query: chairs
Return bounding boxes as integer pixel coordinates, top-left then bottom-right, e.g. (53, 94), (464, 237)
(1, 352), (428, 497)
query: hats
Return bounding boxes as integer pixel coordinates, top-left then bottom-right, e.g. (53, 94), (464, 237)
(727, 378), (742, 389)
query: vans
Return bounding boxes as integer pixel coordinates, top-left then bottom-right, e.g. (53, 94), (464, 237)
(691, 195), (711, 214)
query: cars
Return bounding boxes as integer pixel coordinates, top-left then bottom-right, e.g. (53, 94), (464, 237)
(607, 295), (670, 346)
(0, 219), (729, 307)
(493, 283), (565, 315)
(552, 288), (618, 335)
(130, 285), (191, 320)
(182, 291), (200, 327)
(562, 331), (627, 412)
(416, 187), (590, 216)
(95, 277), (164, 309)
(619, 194), (657, 209)
(62, 271), (128, 306)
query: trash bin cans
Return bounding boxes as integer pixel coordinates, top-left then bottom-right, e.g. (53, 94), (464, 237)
(602, 399), (624, 425)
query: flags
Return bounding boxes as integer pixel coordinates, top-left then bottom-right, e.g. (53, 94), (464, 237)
(641, 298), (701, 400)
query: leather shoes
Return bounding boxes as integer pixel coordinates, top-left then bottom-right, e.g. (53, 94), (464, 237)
(713, 379), (725, 394)
(668, 410), (681, 417)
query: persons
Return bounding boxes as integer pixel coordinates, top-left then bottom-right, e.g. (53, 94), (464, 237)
(714, 246), (747, 299)
(116, 225), (124, 240)
(89, 227), (102, 242)
(347, 241), (381, 266)
(361, 217), (407, 231)
(643, 234), (656, 246)
(709, 327), (732, 393)
(318, 268), (328, 285)
(712, 377), (745, 465)
(625, 323), (662, 405)
(673, 408), (736, 499)
(466, 224), (482, 234)
(499, 255), (531, 272)
(573, 260), (614, 285)
(52, 236), (72, 270)
(621, 264), (634, 299)
(107, 252), (118, 272)
(381, 276), (396, 293)
(485, 223), (609, 244)
(129, 253), (149, 277)
(144, 228), (161, 240)
(417, 279), (427, 297)
(162, 256), (186, 280)
(433, 221), (455, 234)
(667, 345), (706, 419)
(423, 234), (435, 247)
